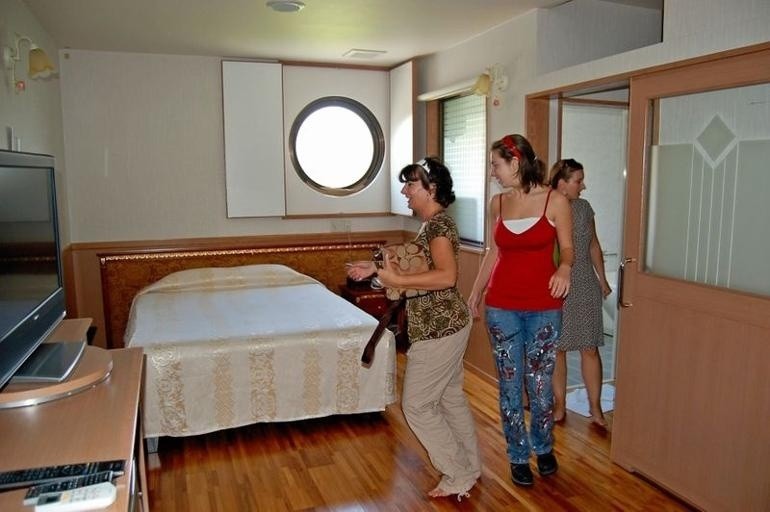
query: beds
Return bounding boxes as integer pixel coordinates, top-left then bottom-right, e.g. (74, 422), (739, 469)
(129, 283), (396, 469)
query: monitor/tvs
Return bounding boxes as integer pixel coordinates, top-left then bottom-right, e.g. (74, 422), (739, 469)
(0, 149), (87, 392)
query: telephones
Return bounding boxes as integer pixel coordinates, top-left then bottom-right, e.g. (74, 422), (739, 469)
(371, 277), (383, 287)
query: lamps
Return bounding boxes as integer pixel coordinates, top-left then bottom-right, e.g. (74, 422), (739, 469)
(266, 0), (305, 13)
(2, 35), (60, 95)
(460, 63), (509, 108)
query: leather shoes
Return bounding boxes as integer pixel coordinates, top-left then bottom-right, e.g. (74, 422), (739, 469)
(536, 451), (558, 476)
(510, 462), (534, 486)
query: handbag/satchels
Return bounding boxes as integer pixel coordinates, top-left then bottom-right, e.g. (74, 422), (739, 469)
(373, 241), (433, 302)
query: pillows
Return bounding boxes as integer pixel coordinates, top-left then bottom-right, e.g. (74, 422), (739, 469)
(148, 263), (298, 291)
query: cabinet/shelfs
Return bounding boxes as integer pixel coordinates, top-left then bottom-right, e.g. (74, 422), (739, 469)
(1, 346), (150, 512)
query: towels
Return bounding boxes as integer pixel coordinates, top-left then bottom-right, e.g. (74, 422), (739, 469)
(566, 382), (616, 418)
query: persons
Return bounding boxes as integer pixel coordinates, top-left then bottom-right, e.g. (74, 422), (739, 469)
(465, 133), (575, 486)
(546, 158), (611, 425)
(344, 157), (480, 497)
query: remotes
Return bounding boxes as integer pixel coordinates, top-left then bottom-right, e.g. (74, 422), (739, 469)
(34, 481), (117, 512)
(0, 459), (127, 491)
(21, 471), (114, 506)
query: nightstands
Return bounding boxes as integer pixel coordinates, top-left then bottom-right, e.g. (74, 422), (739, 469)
(336, 282), (408, 354)
